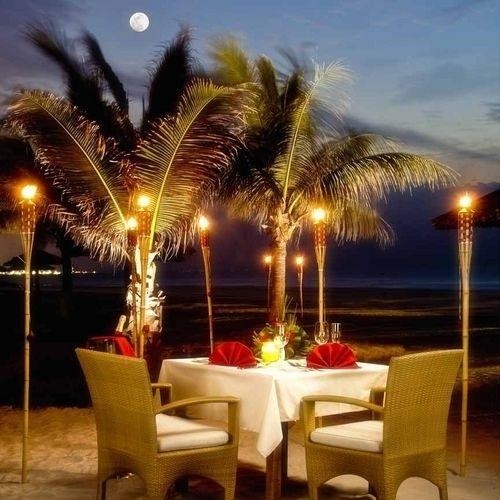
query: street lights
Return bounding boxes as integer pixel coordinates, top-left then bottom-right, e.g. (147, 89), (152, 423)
(14, 183), (44, 484)
(309, 206), (329, 322)
(126, 194), (153, 358)
(450, 191), (480, 480)
(196, 214), (214, 358)
(263, 253), (305, 322)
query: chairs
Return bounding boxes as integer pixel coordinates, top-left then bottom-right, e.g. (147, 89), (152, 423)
(298, 348), (466, 500)
(74, 346), (243, 498)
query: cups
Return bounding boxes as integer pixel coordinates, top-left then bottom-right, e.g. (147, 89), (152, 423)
(331, 323), (341, 343)
(314, 322), (329, 345)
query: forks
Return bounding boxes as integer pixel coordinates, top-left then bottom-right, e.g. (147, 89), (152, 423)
(288, 361), (321, 372)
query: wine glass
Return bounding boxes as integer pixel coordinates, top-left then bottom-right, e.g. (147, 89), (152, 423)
(273, 321), (289, 363)
